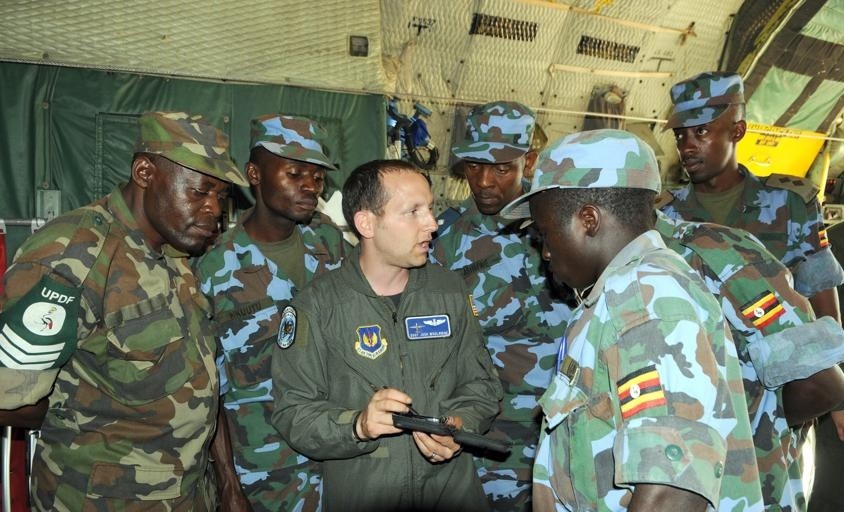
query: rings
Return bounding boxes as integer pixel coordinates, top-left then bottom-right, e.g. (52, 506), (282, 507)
(429, 452), (437, 459)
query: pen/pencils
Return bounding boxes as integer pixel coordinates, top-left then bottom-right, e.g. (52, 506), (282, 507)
(383, 386), (419, 415)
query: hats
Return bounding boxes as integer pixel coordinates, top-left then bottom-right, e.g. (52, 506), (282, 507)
(661, 71), (746, 132)
(249, 112), (336, 171)
(453, 100), (536, 163)
(500, 128), (662, 220)
(134, 108), (249, 188)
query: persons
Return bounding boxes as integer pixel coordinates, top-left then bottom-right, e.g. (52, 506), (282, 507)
(191, 113), (347, 512)
(501, 130), (765, 512)
(271, 158), (503, 511)
(654, 210), (844, 512)
(430, 100), (585, 511)
(660, 71), (839, 329)
(1, 110), (251, 512)
